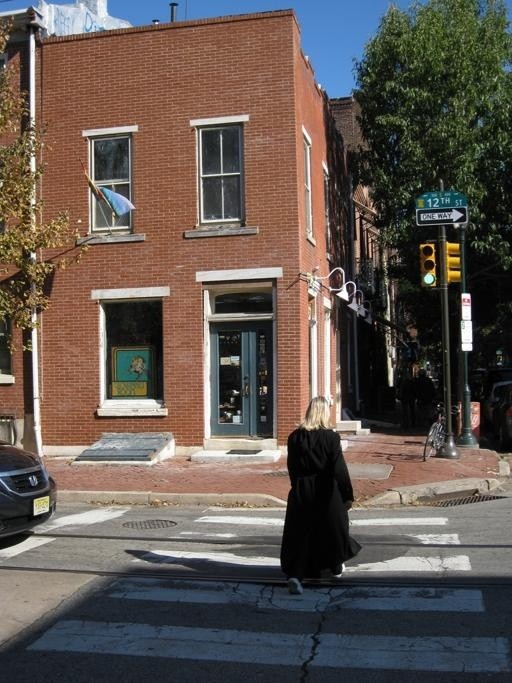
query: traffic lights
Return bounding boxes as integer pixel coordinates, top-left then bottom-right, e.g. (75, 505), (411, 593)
(445, 241), (461, 284)
(418, 242), (437, 288)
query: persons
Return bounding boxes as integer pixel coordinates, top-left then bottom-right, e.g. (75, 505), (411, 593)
(395, 371), (421, 428)
(281, 396), (363, 595)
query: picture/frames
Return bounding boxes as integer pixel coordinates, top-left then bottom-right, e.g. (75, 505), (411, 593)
(110, 346), (157, 399)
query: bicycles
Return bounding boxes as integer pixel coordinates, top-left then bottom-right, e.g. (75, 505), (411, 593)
(422, 401), (455, 460)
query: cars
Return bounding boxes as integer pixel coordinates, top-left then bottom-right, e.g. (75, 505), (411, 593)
(1, 439), (57, 537)
(474, 366), (511, 450)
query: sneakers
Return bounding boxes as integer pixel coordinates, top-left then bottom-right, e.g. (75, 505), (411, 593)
(288, 578), (303, 594)
(331, 563), (345, 578)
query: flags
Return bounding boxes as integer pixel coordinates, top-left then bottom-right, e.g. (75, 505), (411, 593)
(84, 167), (135, 220)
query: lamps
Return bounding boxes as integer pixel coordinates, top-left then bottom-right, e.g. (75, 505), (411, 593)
(306, 266), (373, 325)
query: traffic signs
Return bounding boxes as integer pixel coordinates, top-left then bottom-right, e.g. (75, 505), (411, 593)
(416, 192), (468, 209)
(415, 207), (469, 226)
